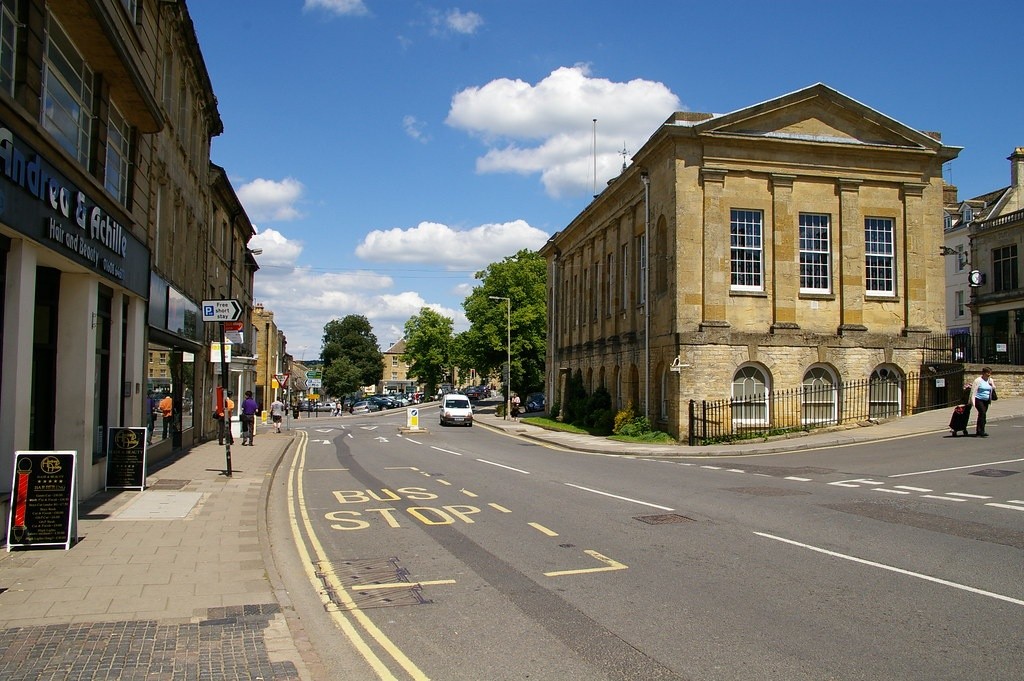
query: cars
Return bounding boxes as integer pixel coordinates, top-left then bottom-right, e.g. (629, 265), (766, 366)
(464, 386), (491, 400)
(365, 392), (425, 411)
(299, 398), (337, 412)
(524, 392), (546, 412)
(352, 401), (380, 415)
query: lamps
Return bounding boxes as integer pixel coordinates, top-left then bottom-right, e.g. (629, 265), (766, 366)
(247, 248), (262, 257)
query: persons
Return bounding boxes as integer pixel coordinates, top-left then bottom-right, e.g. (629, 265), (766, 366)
(511, 393), (520, 417)
(160, 391), (172, 439)
(968, 366), (996, 437)
(271, 397), (284, 433)
(956, 349), (963, 361)
(146, 389), (156, 444)
(241, 390), (258, 446)
(330, 400), (341, 416)
(438, 387), (442, 400)
(411, 393), (419, 404)
(215, 391), (234, 445)
(298, 400), (302, 405)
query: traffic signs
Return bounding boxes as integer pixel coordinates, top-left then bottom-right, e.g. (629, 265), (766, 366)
(304, 379), (322, 388)
(305, 370), (322, 379)
(202, 298), (243, 323)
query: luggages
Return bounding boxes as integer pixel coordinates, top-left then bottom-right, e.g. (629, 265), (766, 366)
(948, 403), (972, 437)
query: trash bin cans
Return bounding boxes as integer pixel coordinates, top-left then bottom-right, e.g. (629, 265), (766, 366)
(293, 405), (299, 419)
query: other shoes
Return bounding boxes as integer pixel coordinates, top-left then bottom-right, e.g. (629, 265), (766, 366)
(219, 444), (225, 445)
(249, 444), (254, 446)
(278, 428), (281, 433)
(976, 432), (989, 436)
(242, 442), (247, 446)
(231, 441), (234, 445)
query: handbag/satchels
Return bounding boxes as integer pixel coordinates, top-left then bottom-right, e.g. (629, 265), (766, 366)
(988, 378), (998, 400)
(514, 404), (519, 410)
(212, 411), (220, 419)
(338, 409), (341, 414)
(153, 413), (157, 421)
(240, 414), (243, 421)
(334, 409), (338, 413)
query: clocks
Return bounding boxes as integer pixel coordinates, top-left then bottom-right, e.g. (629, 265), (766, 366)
(969, 270), (983, 285)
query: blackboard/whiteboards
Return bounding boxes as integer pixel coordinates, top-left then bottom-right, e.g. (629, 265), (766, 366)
(104, 427), (147, 488)
(7, 450), (78, 547)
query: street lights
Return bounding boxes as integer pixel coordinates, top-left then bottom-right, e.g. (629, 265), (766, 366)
(489, 296), (512, 420)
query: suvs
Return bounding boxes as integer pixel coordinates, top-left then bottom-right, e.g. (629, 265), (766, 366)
(439, 394), (475, 427)
